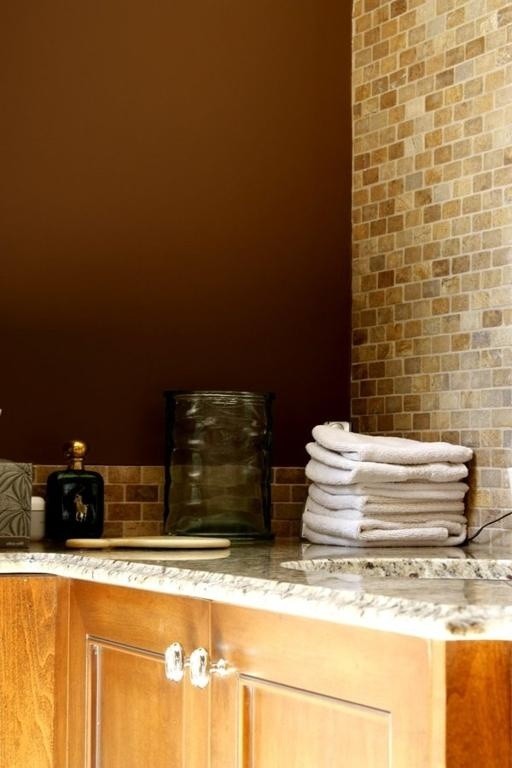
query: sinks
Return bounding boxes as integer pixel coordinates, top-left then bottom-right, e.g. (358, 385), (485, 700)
(280, 557), (512, 580)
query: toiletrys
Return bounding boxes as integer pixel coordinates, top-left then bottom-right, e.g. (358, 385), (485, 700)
(28, 496), (47, 544)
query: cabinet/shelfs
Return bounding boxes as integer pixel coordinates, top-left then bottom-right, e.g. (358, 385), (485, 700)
(51, 576), (511, 768)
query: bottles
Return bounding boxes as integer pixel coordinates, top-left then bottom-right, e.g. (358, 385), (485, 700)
(42, 437), (105, 543)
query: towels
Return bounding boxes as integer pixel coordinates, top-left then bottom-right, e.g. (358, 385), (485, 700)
(300, 424), (475, 548)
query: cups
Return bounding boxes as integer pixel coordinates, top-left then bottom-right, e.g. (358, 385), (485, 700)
(162, 388), (274, 548)
